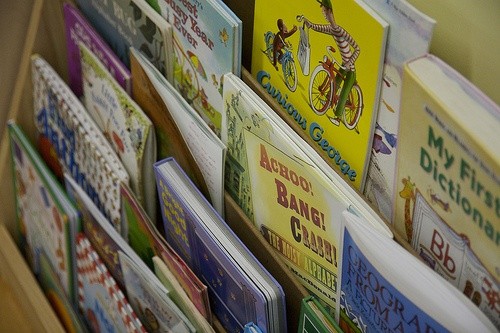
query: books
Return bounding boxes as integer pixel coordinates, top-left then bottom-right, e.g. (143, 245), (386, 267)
(9, 0), (500, 333)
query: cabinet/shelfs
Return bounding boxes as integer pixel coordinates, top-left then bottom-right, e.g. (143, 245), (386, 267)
(0, 0), (500, 333)
